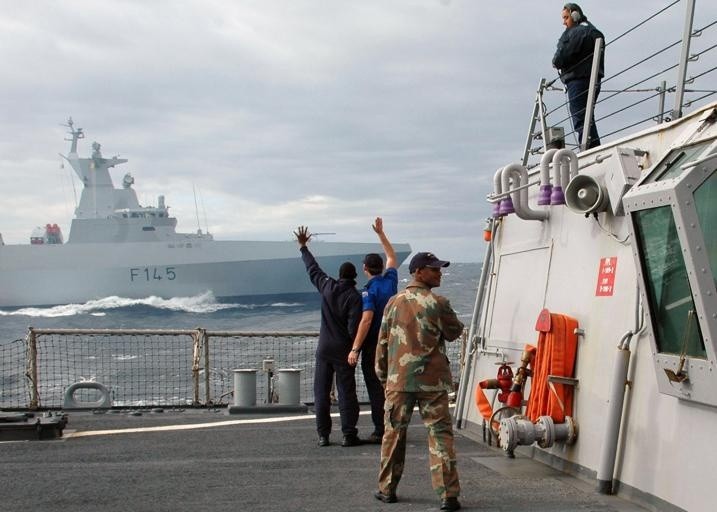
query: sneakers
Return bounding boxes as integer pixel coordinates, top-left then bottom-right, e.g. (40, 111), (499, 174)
(318, 433), (382, 447)
(441, 498), (461, 509)
(374, 490), (398, 502)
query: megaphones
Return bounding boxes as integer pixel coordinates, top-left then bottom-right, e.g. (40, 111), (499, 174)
(564, 175), (609, 214)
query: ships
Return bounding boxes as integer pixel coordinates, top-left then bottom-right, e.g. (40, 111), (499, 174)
(0, 2), (717, 512)
(1, 117), (413, 313)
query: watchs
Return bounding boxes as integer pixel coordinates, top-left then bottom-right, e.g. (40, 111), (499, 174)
(351, 349), (358, 352)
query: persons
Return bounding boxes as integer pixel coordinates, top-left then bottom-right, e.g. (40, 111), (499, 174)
(375, 253), (464, 510)
(294, 226), (361, 446)
(348, 217), (397, 437)
(552, 3), (604, 152)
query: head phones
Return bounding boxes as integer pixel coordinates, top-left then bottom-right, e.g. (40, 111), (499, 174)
(564, 4), (580, 22)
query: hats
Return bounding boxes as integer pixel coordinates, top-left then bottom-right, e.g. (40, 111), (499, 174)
(409, 252), (451, 274)
